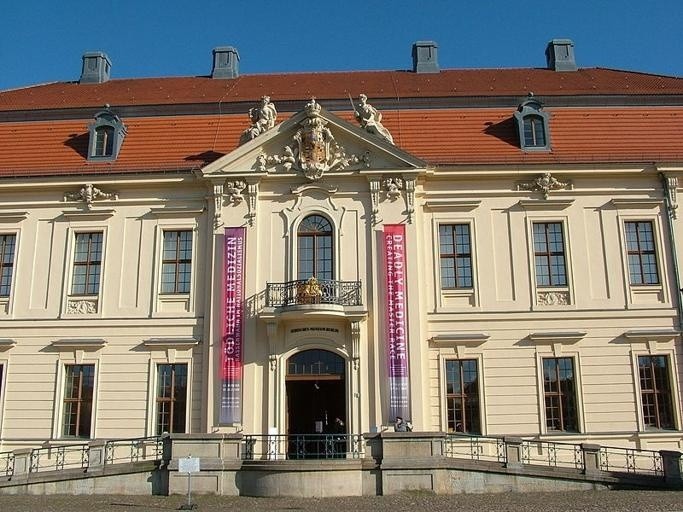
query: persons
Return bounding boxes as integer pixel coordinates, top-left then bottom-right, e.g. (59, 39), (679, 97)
(236, 95), (277, 146)
(354, 93), (395, 147)
(395, 416), (411, 432)
(335, 415), (346, 458)
